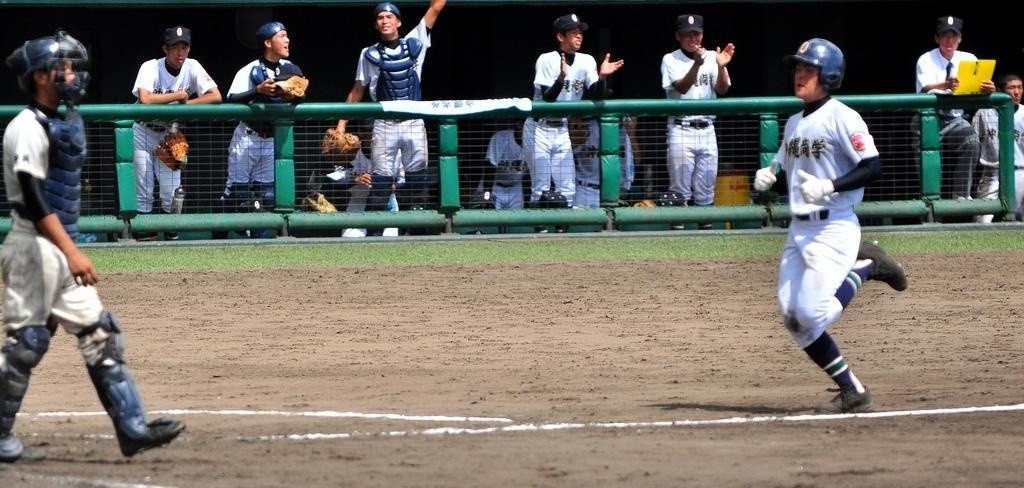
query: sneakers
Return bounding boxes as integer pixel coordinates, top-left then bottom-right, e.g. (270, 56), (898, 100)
(699, 221), (713, 230)
(166, 231), (179, 240)
(671, 222), (685, 230)
(0, 436), (47, 463)
(855, 241), (908, 292)
(555, 224), (566, 232)
(114, 416), (186, 458)
(815, 385), (875, 415)
(133, 231), (158, 241)
(534, 226), (548, 234)
(952, 190), (973, 200)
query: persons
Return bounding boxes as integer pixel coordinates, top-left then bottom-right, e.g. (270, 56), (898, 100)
(753, 37), (908, 415)
(523, 14), (625, 234)
(209, 23), (307, 239)
(321, 0), (445, 237)
(475, 118), (529, 209)
(566, 87), (636, 210)
(620, 116), (642, 208)
(660, 14), (735, 230)
(132, 27), (221, 236)
(1, 31), (186, 459)
(910, 15), (996, 225)
(971, 74), (1024, 224)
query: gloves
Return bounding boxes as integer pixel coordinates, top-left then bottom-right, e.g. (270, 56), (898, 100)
(753, 158), (780, 192)
(794, 169), (834, 205)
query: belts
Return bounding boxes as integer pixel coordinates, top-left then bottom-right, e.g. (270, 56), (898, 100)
(788, 205), (857, 223)
(494, 180), (517, 188)
(983, 164), (1024, 170)
(672, 119), (713, 130)
(578, 180), (600, 190)
(245, 128), (273, 139)
(533, 118), (568, 130)
(136, 118), (174, 133)
(392, 118), (403, 122)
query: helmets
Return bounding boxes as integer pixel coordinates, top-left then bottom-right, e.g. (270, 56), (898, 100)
(254, 21), (287, 42)
(373, 1), (401, 18)
(785, 37), (847, 90)
(7, 31), (89, 106)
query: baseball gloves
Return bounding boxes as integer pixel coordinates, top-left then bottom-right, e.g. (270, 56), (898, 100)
(152, 132), (189, 169)
(272, 75), (308, 98)
(322, 130), (361, 161)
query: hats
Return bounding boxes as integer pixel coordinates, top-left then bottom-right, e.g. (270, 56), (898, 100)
(937, 15), (963, 35)
(677, 14), (704, 36)
(552, 13), (588, 34)
(164, 25), (191, 46)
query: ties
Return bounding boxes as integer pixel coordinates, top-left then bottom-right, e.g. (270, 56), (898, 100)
(946, 61), (953, 81)
